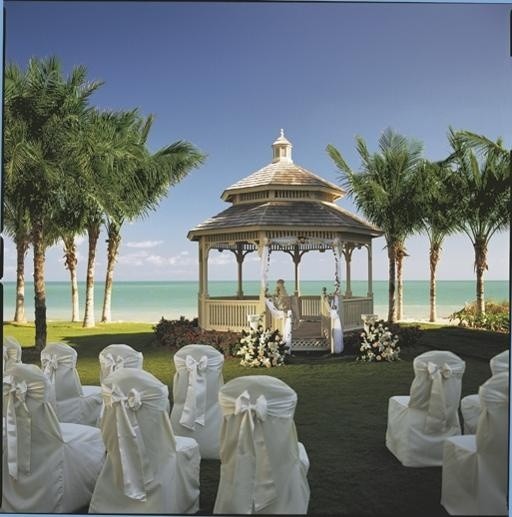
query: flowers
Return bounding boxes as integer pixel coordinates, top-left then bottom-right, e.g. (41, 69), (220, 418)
(356, 320), (400, 363)
(237, 324), (293, 369)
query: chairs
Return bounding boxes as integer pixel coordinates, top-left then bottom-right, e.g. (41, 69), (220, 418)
(386, 347), (508, 516)
(212, 371), (313, 516)
(89, 368), (201, 515)
(169, 345), (226, 461)
(1, 339), (22, 363)
(99, 342), (144, 376)
(4, 363), (108, 513)
(44, 340), (103, 426)
